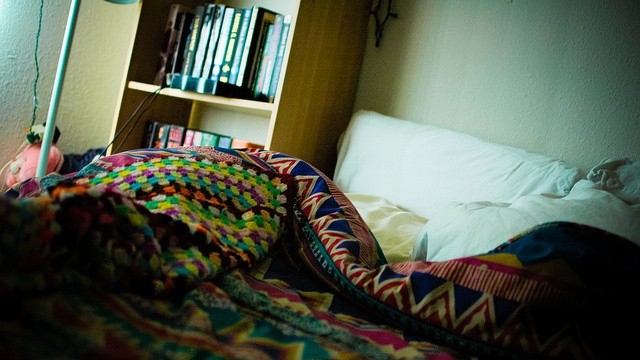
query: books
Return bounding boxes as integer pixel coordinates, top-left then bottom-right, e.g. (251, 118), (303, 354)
(153, 2), (293, 104)
(141, 118), (265, 152)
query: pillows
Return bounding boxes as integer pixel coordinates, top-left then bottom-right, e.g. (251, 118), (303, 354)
(332, 107), (583, 221)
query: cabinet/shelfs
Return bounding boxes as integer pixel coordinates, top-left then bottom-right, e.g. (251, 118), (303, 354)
(105, 1), (371, 181)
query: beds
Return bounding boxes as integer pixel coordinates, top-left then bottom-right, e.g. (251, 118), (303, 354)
(0, 108), (640, 359)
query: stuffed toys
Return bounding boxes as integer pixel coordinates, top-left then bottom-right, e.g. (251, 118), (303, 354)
(5, 121), (64, 188)
